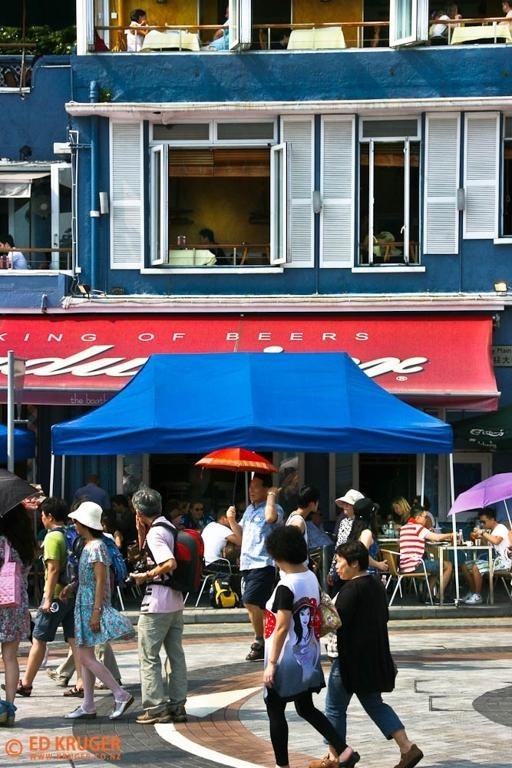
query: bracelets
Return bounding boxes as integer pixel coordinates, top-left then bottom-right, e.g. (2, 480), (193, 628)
(44, 599), (51, 604)
(93, 607), (102, 612)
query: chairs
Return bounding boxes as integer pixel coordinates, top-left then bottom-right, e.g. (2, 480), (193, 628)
(117, 31), (126, 52)
(379, 543), (402, 598)
(480, 553), (512, 605)
(183, 556), (232, 607)
(371, 25), (379, 47)
(259, 28), (268, 50)
(380, 549), (434, 606)
(239, 241), (248, 265)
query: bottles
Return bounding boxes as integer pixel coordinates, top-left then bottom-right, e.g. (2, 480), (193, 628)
(389, 521), (394, 538)
(181, 236), (188, 249)
(175, 236), (182, 250)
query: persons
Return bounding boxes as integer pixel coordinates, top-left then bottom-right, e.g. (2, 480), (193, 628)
(196, 228), (230, 264)
(45, 641), (125, 687)
(0, 231), (28, 271)
(271, 30), (289, 51)
(174, 500), (213, 530)
(128, 8), (153, 52)
(460, 506), (512, 604)
(130, 488), (189, 724)
(201, 505), (240, 596)
(328, 489), (366, 599)
(362, 224), (408, 263)
(493, 3), (512, 44)
(260, 527), (360, 768)
(1, 497), (86, 698)
(99, 511), (126, 605)
(72, 472), (113, 516)
(228, 469), (285, 661)
(213, 7), (229, 49)
(309, 539), (423, 768)
(350, 499), (387, 580)
(431, 5), (458, 46)
(400, 506), (455, 606)
(114, 495), (136, 523)
(390, 496), (413, 528)
(280, 487), (319, 580)
(65, 501), (136, 718)
(0, 503), (35, 725)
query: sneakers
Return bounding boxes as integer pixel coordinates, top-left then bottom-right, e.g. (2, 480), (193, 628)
(394, 744), (423, 768)
(461, 593), (472, 603)
(308, 755), (339, 768)
(465, 594), (483, 605)
(46, 668), (69, 687)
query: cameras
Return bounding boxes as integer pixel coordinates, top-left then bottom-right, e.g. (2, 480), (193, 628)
(125, 560), (150, 588)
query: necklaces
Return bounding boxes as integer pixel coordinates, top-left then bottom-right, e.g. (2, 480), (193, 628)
(282, 568), (309, 572)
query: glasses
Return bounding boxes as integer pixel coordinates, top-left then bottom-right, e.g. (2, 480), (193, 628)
(478, 518), (493, 524)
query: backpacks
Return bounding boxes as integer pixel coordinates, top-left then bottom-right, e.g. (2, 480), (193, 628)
(207, 574), (238, 608)
(47, 526), (87, 585)
(101, 534), (128, 594)
(145, 523), (205, 594)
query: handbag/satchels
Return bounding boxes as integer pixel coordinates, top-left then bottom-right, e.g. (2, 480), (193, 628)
(0, 539), (21, 608)
(321, 592), (341, 639)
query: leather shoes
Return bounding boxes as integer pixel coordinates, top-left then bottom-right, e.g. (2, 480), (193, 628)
(169, 707), (186, 721)
(136, 709), (170, 724)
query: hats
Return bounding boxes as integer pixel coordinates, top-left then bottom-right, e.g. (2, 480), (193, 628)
(67, 502), (103, 530)
(335, 489), (364, 508)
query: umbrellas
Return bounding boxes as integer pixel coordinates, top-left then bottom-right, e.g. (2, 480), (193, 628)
(48, 354), (460, 607)
(447, 471), (512, 531)
(0, 424), (33, 467)
(0, 467), (41, 523)
(196, 446), (277, 508)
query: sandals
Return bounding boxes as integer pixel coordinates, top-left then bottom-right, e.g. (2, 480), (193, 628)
(0, 700), (16, 726)
(65, 705), (97, 719)
(1, 680), (32, 697)
(338, 751), (361, 768)
(63, 686), (85, 698)
(109, 694), (134, 720)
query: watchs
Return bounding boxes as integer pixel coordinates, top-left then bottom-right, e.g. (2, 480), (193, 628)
(266, 492), (276, 497)
(145, 570), (151, 581)
